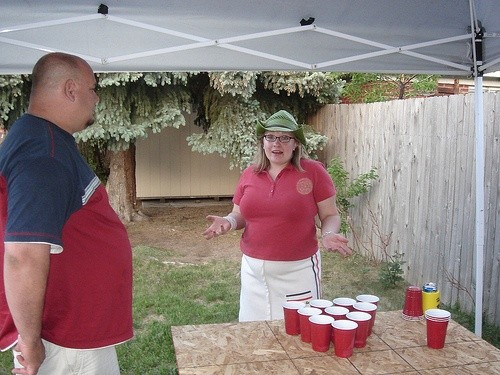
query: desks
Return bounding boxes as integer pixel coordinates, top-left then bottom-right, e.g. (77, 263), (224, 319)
(171, 309), (500, 375)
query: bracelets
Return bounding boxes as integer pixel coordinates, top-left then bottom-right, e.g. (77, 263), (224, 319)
(321, 231), (332, 238)
(222, 216), (237, 233)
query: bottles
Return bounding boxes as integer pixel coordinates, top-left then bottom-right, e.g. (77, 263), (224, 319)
(422, 283), (440, 315)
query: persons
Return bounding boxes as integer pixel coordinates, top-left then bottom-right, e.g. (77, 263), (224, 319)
(0, 52), (134, 375)
(202, 109), (354, 323)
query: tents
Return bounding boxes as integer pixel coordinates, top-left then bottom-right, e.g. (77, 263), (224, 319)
(0, 0), (500, 341)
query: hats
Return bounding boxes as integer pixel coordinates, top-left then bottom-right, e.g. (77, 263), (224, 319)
(256, 109), (306, 146)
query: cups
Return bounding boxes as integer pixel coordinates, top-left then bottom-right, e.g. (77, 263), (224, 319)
(401, 286), (424, 322)
(333, 297), (356, 312)
(425, 309), (451, 349)
(309, 299), (334, 315)
(308, 316), (335, 352)
(297, 307), (322, 343)
(345, 311), (372, 349)
(331, 320), (358, 358)
(353, 302), (377, 336)
(324, 306), (349, 341)
(356, 294), (380, 305)
(281, 301), (307, 336)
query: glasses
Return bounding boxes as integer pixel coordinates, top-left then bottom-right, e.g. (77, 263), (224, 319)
(264, 134), (295, 143)
